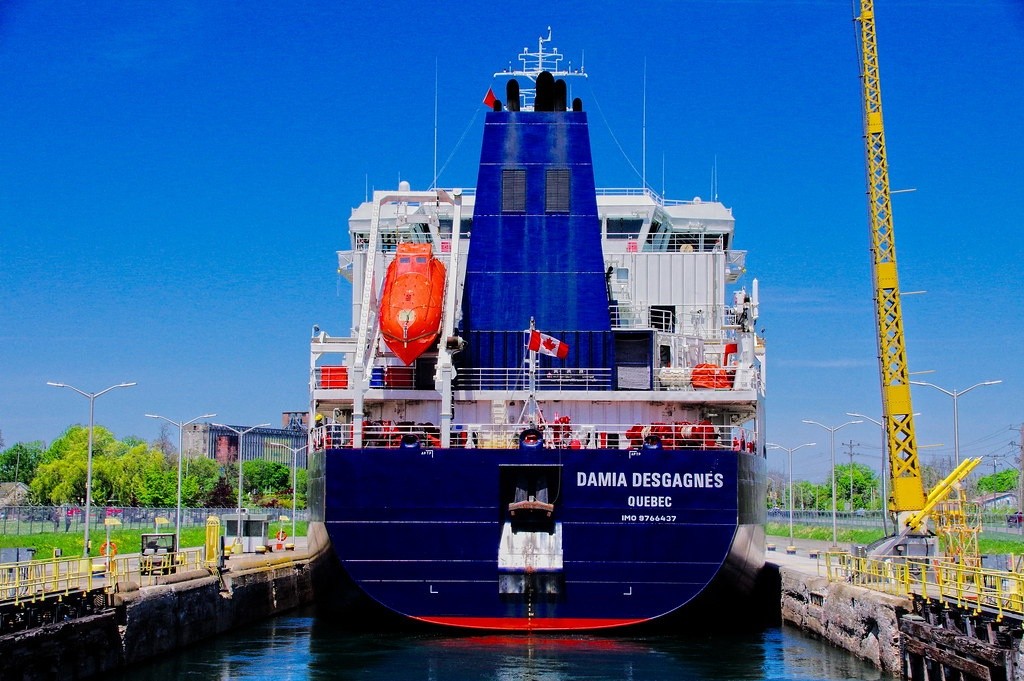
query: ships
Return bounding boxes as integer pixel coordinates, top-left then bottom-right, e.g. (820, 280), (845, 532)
(302, 23), (771, 640)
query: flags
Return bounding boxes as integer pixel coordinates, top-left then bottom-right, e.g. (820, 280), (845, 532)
(528, 329), (569, 359)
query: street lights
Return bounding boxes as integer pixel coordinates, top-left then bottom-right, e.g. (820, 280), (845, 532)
(845, 411), (921, 541)
(764, 441), (817, 547)
(271, 442), (308, 549)
(144, 412), (218, 563)
(46, 381), (139, 570)
(800, 419), (863, 549)
(210, 422), (270, 553)
(909, 380), (1004, 468)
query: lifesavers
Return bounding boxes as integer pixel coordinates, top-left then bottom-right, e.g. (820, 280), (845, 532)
(399, 435), (421, 449)
(100, 541), (118, 559)
(643, 434), (663, 450)
(519, 428), (544, 450)
(274, 530), (288, 542)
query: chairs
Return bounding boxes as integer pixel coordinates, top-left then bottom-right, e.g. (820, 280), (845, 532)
(144, 548), (155, 556)
(158, 548), (167, 555)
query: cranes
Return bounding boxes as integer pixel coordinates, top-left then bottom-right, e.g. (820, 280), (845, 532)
(846, 0), (985, 581)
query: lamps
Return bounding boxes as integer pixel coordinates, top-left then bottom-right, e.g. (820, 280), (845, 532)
(336, 410), (343, 417)
